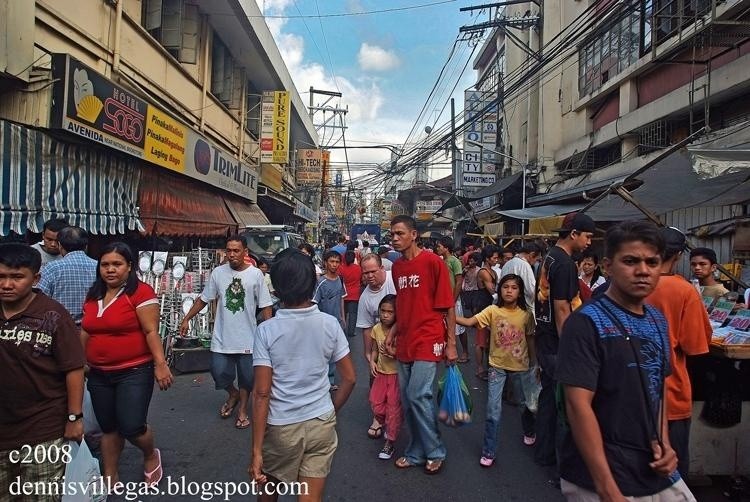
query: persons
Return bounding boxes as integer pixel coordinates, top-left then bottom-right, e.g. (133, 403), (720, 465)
(78, 241), (174, 496)
(643, 225), (713, 478)
(72, 63), (94, 116)
(30, 218), (71, 271)
(36, 226), (99, 329)
(383, 214), (458, 475)
(248, 248), (357, 502)
(177, 211), (609, 467)
(688, 248), (731, 296)
(0, 243), (88, 502)
(554, 219), (697, 502)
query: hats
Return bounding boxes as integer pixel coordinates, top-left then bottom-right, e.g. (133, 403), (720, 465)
(552, 212), (597, 235)
(663, 227), (687, 249)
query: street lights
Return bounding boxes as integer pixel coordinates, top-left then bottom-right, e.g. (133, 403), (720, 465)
(463, 138), (529, 251)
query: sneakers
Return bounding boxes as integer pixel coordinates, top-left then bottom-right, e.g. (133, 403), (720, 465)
(479, 453), (495, 468)
(522, 434), (537, 446)
(378, 444), (395, 459)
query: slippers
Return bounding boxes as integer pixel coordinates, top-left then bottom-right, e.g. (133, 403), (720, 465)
(143, 449), (164, 489)
(424, 457), (446, 475)
(236, 413), (251, 429)
(395, 454), (419, 468)
(458, 356), (472, 364)
(219, 397), (239, 419)
(106, 474), (120, 492)
(367, 426), (382, 438)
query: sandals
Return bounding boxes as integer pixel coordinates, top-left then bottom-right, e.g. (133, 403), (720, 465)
(474, 370), (489, 381)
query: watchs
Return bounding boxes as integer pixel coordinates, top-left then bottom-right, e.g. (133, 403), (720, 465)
(67, 412), (85, 422)
